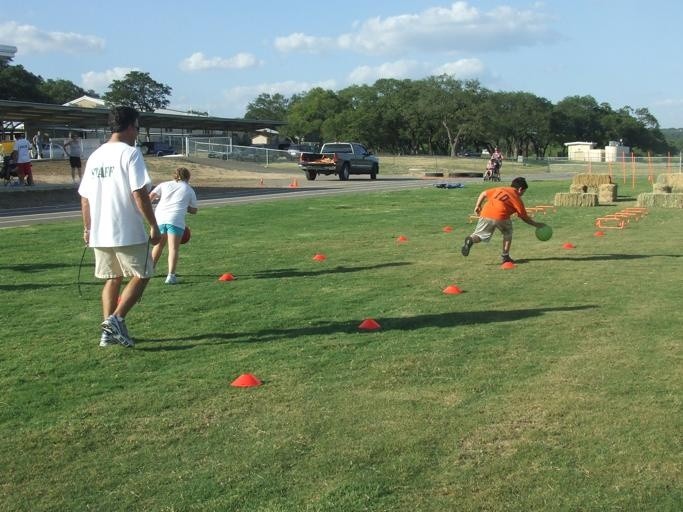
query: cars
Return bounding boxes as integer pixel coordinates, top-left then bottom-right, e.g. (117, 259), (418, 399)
(456, 150), (480, 157)
(209, 142), (317, 161)
(30, 144), (66, 159)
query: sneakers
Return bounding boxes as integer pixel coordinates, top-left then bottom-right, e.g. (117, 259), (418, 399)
(165, 273), (179, 284)
(462, 236), (473, 257)
(501, 254), (514, 264)
(99, 314), (134, 347)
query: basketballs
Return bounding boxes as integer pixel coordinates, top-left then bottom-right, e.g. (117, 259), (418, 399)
(180, 227), (190, 244)
(535, 225), (553, 241)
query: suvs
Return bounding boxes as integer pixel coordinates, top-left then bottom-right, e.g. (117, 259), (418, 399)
(140, 142), (178, 157)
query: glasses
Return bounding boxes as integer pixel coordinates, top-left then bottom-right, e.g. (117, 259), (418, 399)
(135, 126), (139, 130)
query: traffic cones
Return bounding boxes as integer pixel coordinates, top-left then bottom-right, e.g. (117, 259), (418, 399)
(260, 177), (264, 185)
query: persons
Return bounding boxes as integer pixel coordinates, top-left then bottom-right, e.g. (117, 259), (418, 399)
(12, 132), (34, 186)
(482, 160), (493, 179)
(0, 151), (32, 186)
(491, 147), (503, 182)
(77, 105), (161, 350)
(62, 131), (83, 184)
(32, 130), (43, 159)
(149, 165), (198, 285)
(461, 177), (547, 264)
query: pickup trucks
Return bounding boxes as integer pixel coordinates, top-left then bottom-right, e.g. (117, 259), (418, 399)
(300, 142), (379, 181)
